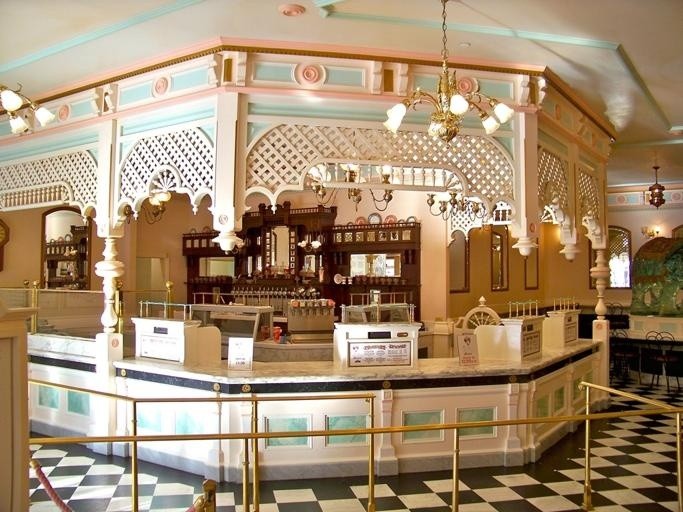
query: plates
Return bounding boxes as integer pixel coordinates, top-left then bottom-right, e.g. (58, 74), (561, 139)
(332, 212), (419, 229)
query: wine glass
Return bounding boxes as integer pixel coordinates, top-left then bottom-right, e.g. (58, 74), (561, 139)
(353, 273), (408, 287)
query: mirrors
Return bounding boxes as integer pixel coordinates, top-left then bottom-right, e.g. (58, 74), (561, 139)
(39, 206), (91, 292)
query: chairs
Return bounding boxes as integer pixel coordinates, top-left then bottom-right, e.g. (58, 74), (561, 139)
(604, 302), (628, 327)
(607, 328), (681, 396)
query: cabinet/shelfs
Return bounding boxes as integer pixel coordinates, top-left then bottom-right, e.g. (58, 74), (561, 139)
(181, 205), (423, 326)
(46, 224), (88, 287)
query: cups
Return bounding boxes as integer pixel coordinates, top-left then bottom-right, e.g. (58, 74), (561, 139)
(260, 326), (269, 340)
(271, 327), (282, 343)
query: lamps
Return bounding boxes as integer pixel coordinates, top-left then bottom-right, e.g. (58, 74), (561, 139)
(382, 0), (516, 148)
(0, 85), (56, 140)
(647, 166), (667, 211)
(306, 131), (396, 211)
(125, 193), (172, 225)
(426, 192), (497, 223)
